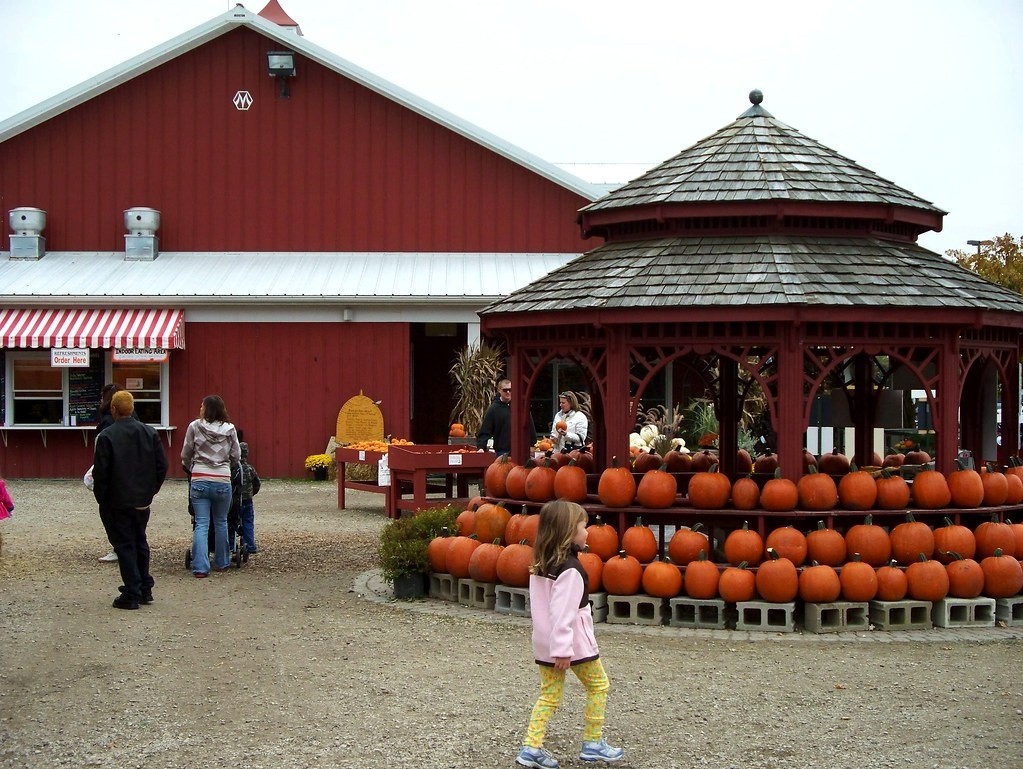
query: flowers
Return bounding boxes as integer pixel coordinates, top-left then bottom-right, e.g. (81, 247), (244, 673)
(303, 453), (332, 471)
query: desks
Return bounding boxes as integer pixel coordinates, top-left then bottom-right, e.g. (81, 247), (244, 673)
(335, 446), (390, 518)
(388, 444), (496, 519)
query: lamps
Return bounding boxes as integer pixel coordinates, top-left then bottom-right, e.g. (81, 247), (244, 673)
(266, 51), (296, 98)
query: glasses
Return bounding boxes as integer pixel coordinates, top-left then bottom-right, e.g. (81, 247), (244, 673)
(562, 392), (570, 403)
(501, 389), (512, 392)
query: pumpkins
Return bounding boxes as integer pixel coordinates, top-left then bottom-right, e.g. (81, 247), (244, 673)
(345, 439), (414, 452)
(426, 418), (1023, 601)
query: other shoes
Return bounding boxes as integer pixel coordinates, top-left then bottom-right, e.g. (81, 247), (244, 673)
(113, 596), (138, 610)
(195, 572), (206, 578)
(118, 585), (153, 603)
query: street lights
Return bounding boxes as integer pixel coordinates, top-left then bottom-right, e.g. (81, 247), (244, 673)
(967, 239), (995, 273)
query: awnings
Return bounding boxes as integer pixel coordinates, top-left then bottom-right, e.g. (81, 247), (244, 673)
(0, 308), (185, 349)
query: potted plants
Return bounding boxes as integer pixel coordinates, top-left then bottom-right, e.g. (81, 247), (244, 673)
(378, 505), (462, 599)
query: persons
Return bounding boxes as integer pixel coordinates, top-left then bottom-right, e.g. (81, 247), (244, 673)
(0, 480), (14, 557)
(630, 397), (648, 434)
(477, 380), (537, 459)
(93, 383), (169, 610)
(550, 391), (588, 454)
(181, 395), (261, 578)
(515, 501), (623, 769)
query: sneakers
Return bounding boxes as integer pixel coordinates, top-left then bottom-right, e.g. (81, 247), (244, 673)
(98, 551), (119, 562)
(579, 738), (623, 762)
(515, 746), (559, 769)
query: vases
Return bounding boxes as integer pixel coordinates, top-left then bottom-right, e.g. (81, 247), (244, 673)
(314, 466), (328, 480)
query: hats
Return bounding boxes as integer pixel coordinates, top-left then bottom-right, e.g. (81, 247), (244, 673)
(238, 441), (249, 457)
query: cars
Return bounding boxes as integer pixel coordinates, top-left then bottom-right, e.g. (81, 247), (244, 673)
(996, 402), (1023, 434)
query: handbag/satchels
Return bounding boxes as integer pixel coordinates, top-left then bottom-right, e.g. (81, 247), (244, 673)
(565, 432), (591, 454)
(84, 463), (95, 491)
